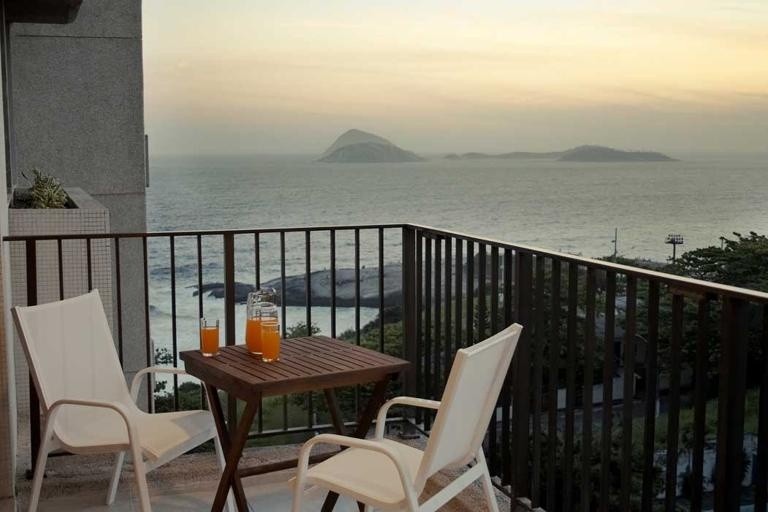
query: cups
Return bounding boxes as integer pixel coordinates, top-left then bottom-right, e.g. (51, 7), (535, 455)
(200, 317), (220, 357)
(245, 289), (279, 355)
(260, 321), (281, 363)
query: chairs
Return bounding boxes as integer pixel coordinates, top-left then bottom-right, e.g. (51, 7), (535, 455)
(11, 288), (236, 512)
(292, 323), (524, 510)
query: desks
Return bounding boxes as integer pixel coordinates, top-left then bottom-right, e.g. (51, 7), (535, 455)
(179, 335), (411, 512)
